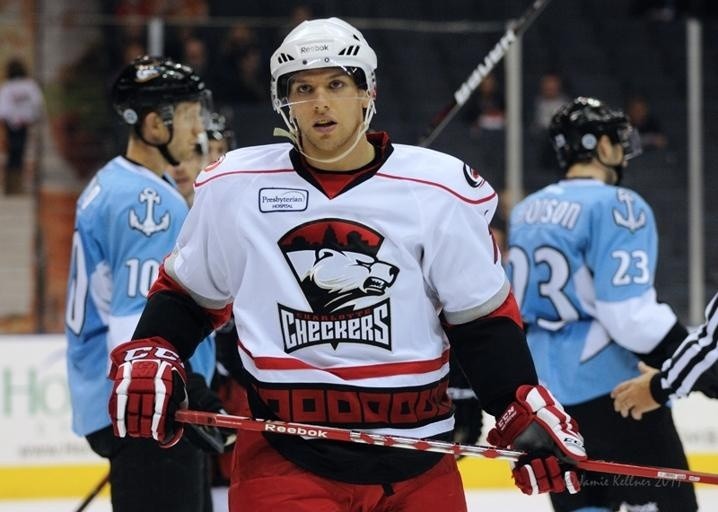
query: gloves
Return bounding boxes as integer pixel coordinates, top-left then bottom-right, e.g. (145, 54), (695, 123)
(485, 385), (587, 495)
(108, 337), (236, 455)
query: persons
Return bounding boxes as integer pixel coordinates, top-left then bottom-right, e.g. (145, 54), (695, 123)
(610, 290), (718, 422)
(454, 66), (667, 214)
(507, 97), (718, 512)
(1, 56), (46, 197)
(67, 56), (225, 508)
(109, 16), (588, 512)
(120, 4), (313, 201)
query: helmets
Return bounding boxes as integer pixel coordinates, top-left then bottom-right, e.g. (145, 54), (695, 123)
(541, 97), (643, 175)
(269, 18), (378, 111)
(111, 52), (235, 171)
